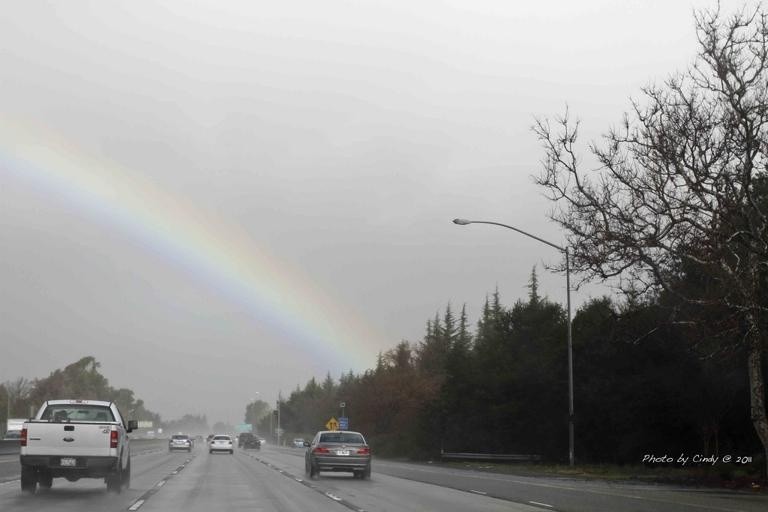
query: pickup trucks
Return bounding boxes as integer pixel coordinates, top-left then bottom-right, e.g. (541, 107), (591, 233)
(19, 396), (137, 495)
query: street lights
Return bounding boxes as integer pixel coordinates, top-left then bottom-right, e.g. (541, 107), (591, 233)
(452, 216), (577, 474)
(255, 391), (273, 443)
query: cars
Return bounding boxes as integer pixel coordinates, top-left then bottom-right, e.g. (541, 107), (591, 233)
(303, 429), (374, 481)
(168, 431), (267, 455)
(291, 438), (307, 448)
